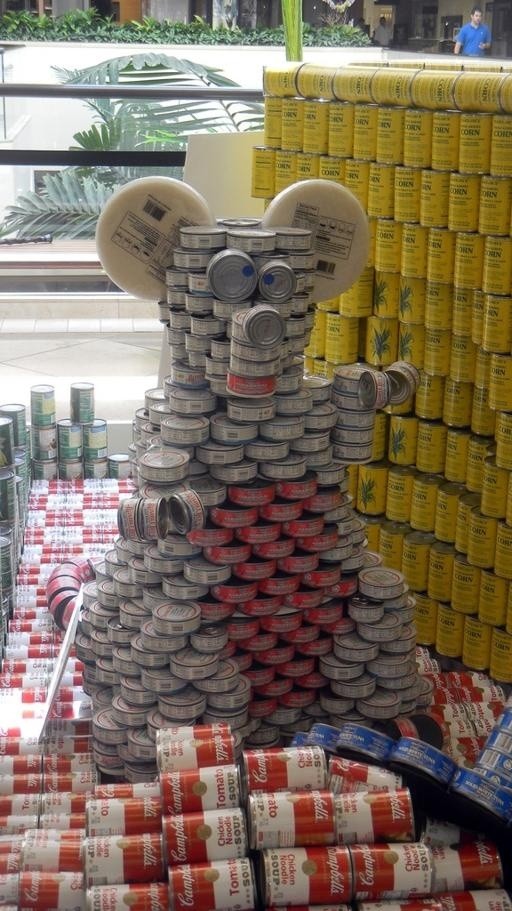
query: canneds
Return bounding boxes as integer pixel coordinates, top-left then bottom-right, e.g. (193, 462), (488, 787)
(0, 59), (512, 911)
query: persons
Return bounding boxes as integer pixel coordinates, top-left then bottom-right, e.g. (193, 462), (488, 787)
(453, 7), (492, 56)
(372, 17), (393, 47)
(348, 16), (365, 30)
